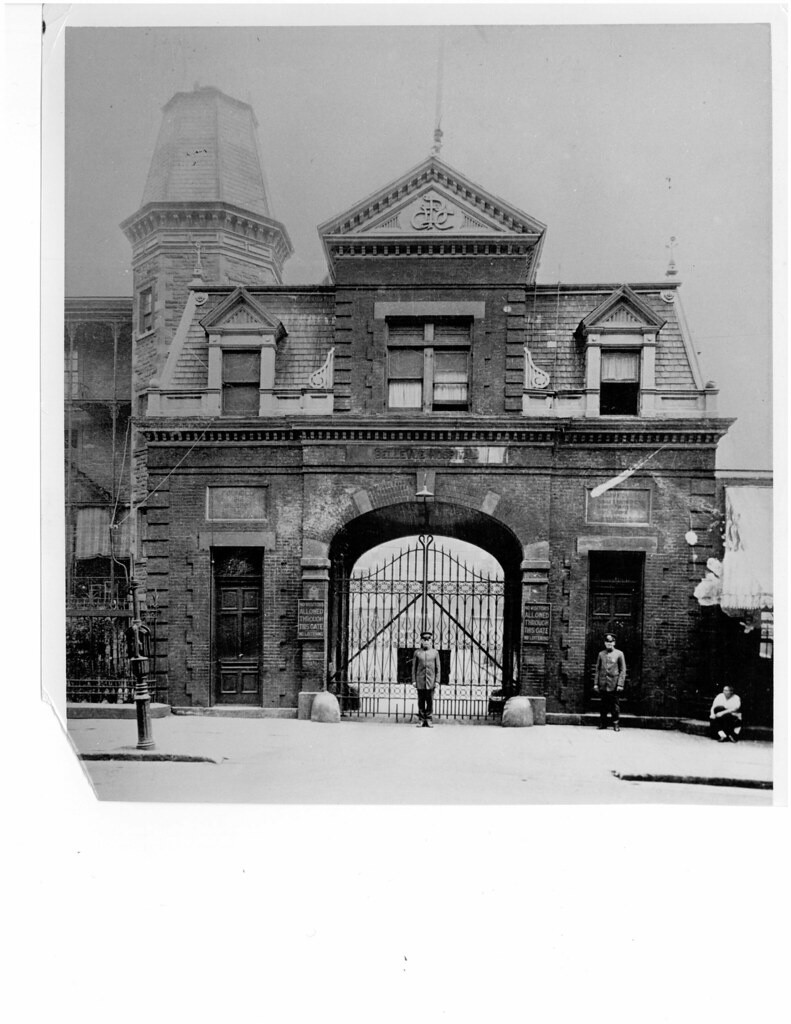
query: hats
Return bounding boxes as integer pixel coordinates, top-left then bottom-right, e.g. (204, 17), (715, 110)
(602, 633), (617, 642)
(420, 632), (433, 639)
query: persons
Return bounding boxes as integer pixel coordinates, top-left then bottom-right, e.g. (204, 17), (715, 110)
(594, 633), (627, 731)
(709, 685), (742, 743)
(411, 633), (441, 727)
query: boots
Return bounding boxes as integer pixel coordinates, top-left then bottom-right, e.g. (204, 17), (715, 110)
(613, 720), (620, 731)
(597, 721), (607, 729)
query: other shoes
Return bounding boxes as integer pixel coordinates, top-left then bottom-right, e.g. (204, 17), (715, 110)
(417, 718), (432, 727)
(718, 735), (738, 742)
